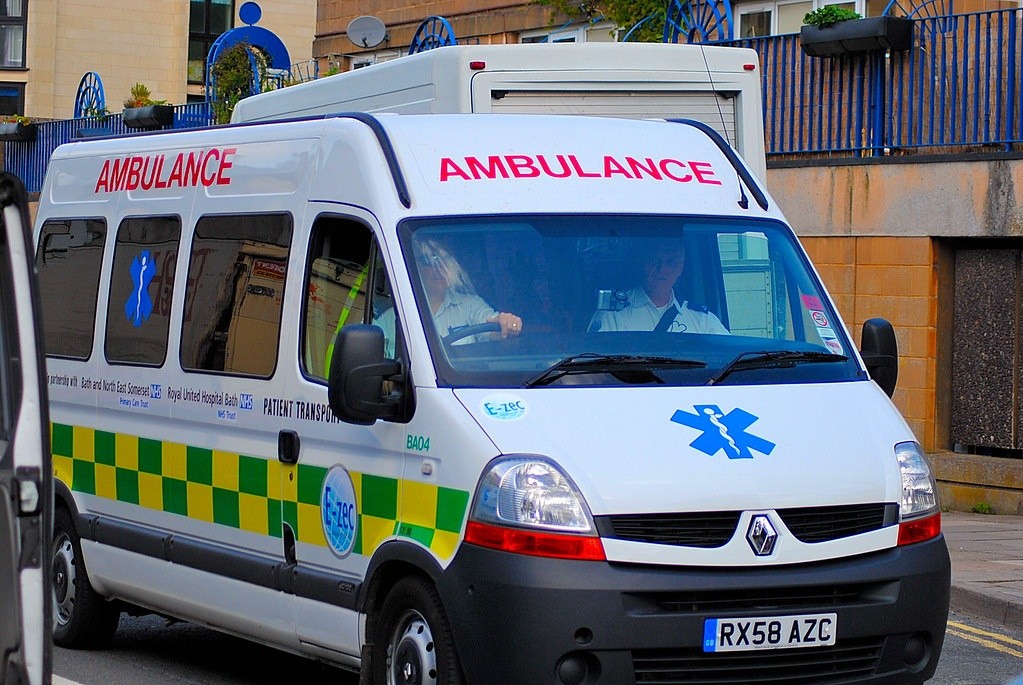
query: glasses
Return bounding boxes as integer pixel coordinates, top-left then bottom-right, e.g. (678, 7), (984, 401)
(417, 255), (438, 266)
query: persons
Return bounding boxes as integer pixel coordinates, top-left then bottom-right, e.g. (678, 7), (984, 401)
(371, 237), (523, 397)
(587, 235), (731, 335)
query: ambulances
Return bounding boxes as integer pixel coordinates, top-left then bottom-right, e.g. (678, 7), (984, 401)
(32, 110), (952, 685)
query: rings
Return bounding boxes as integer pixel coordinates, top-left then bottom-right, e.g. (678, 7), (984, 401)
(512, 324), (516, 327)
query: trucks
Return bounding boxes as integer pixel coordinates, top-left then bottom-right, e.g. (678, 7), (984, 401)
(228, 43), (768, 196)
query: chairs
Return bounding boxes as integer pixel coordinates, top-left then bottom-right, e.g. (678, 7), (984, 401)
(579, 238), (690, 324)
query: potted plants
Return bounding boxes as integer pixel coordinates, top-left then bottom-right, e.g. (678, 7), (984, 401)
(0, 113), (35, 142)
(120, 83), (173, 128)
(800, 4), (915, 57)
